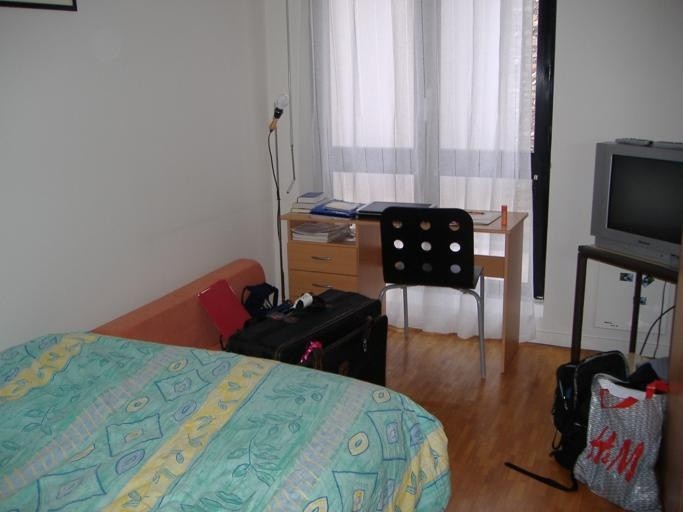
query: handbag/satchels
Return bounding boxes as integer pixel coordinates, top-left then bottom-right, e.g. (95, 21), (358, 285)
(574, 373), (670, 511)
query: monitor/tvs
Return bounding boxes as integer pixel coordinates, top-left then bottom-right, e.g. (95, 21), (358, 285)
(587, 140), (683, 271)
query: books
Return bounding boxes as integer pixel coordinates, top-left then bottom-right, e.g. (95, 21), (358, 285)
(292, 222), (350, 243)
(470, 211), (502, 225)
(312, 200), (365, 218)
(290, 208), (311, 214)
(292, 203), (319, 208)
(297, 192), (324, 203)
(325, 201), (359, 212)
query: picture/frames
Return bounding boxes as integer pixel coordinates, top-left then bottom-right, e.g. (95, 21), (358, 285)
(0, 0), (80, 14)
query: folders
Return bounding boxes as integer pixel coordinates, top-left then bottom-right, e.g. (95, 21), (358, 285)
(355, 201), (437, 215)
(310, 199), (365, 216)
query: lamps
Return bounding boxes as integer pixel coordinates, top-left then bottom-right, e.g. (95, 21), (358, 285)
(266, 94), (291, 302)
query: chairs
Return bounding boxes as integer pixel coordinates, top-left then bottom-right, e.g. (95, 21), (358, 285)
(376, 205), (488, 381)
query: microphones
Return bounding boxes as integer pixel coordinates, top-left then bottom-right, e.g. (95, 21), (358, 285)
(269, 94), (289, 132)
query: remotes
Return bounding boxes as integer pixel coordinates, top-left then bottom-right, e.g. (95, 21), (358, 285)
(648, 139), (682, 150)
(614, 136), (653, 145)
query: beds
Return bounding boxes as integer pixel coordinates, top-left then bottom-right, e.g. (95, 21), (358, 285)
(2, 326), (453, 512)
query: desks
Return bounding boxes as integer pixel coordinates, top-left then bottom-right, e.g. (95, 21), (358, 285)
(277, 206), (530, 375)
(570, 245), (680, 369)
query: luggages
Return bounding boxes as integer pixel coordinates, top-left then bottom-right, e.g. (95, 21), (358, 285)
(224, 287), (388, 386)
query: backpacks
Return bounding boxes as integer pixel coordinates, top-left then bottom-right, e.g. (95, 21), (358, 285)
(504, 349), (631, 491)
